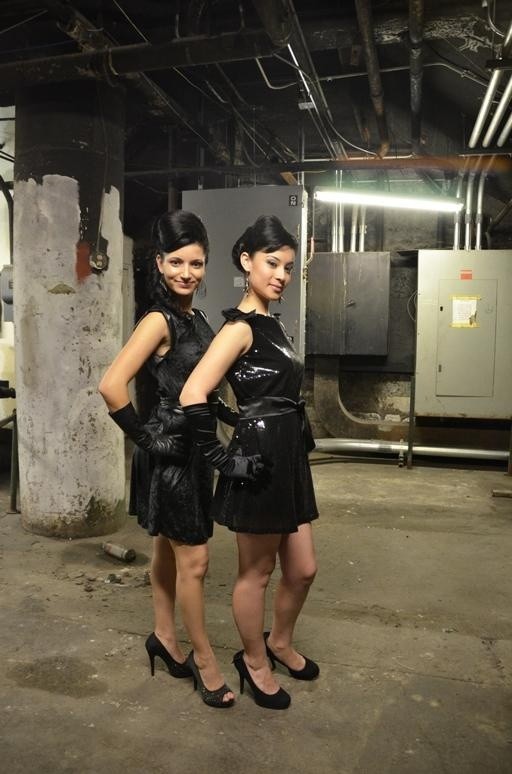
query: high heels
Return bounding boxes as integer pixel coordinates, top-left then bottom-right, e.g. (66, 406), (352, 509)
(145, 632), (191, 678)
(233, 650), (290, 708)
(188, 650), (235, 708)
(262, 631), (319, 681)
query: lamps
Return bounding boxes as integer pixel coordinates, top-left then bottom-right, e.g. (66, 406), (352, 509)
(312, 138), (468, 213)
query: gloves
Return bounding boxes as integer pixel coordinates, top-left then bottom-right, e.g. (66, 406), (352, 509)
(184, 404), (263, 481)
(109, 402), (186, 460)
(215, 395), (238, 428)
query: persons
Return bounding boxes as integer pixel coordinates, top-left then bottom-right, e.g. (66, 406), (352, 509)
(179, 214), (323, 709)
(93, 206), (238, 709)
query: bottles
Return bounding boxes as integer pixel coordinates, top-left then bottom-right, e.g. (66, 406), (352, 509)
(102, 540), (136, 561)
